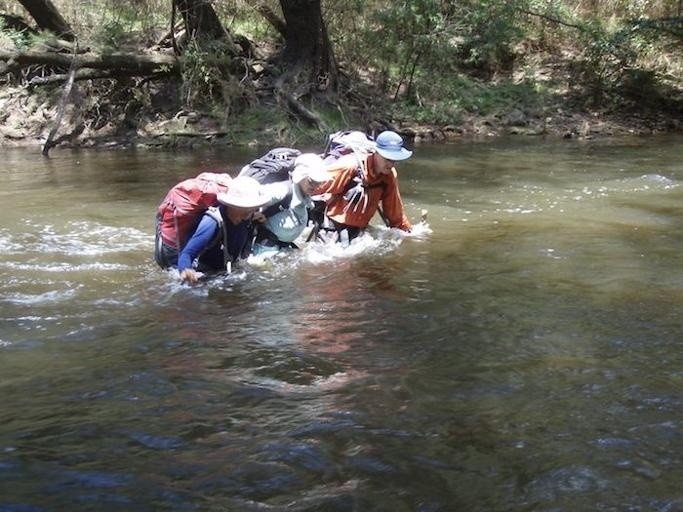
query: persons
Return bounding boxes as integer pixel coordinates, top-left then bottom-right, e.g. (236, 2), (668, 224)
(318, 130), (418, 244)
(253, 148), (333, 255)
(176, 175), (271, 281)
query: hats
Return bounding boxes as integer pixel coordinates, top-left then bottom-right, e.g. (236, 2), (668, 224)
(374, 131), (414, 160)
(217, 176), (271, 208)
(291, 153), (332, 184)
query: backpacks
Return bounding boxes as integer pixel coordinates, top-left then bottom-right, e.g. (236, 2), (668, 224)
(317, 130), (377, 182)
(236, 146), (302, 184)
(155, 172), (233, 271)
